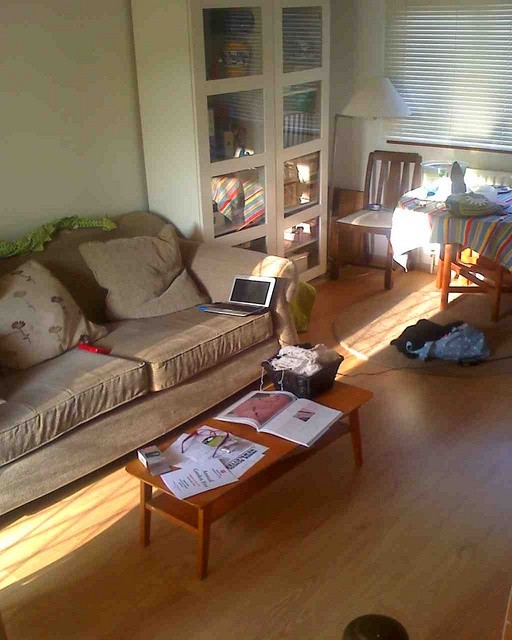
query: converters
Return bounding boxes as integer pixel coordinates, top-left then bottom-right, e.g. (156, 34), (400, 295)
(458, 357), (480, 366)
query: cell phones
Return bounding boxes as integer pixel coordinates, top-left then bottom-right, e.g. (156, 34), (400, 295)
(79, 344), (111, 354)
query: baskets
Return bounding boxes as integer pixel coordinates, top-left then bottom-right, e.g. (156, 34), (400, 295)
(259, 341), (345, 400)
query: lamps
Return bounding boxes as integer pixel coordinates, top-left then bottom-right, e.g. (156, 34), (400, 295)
(339, 74), (415, 279)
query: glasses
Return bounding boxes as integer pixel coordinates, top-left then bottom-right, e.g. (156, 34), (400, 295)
(182, 429), (229, 456)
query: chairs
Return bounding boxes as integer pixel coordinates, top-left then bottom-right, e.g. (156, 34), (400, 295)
(337, 150), (423, 289)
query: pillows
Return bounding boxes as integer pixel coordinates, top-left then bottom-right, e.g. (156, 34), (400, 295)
(1, 258), (111, 371)
(78, 223), (212, 319)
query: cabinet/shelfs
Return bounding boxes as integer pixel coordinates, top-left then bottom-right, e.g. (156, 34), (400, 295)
(130, 1), (330, 284)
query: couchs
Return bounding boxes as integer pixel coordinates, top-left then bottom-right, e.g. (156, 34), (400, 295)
(1, 212), (320, 516)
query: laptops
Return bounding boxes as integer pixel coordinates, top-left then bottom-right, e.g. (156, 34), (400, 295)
(199, 275), (277, 316)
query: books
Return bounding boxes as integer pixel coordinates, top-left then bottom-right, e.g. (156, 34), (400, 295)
(213, 390), (344, 449)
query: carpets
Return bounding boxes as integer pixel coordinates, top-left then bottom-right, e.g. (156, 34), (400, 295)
(331, 286), (512, 379)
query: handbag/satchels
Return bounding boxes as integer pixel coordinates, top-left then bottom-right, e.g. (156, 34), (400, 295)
(435, 191), (504, 217)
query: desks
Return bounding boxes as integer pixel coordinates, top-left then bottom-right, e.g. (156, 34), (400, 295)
(389, 162), (511, 320)
